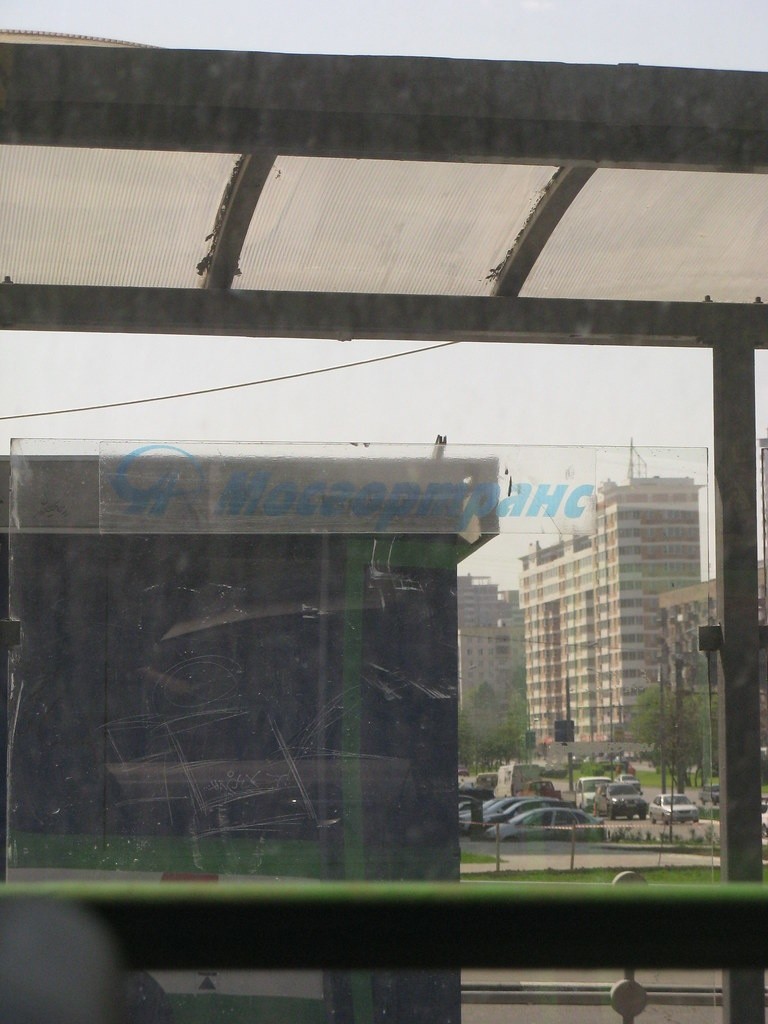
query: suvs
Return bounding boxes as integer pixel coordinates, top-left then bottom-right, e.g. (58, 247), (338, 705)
(491, 763), (542, 798)
(594, 780), (649, 821)
(575, 777), (611, 811)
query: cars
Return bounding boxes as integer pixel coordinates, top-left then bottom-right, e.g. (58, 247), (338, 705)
(457, 772), (586, 840)
(650, 794), (699, 825)
(700, 782), (720, 804)
(604, 751), (634, 783)
(485, 808), (608, 846)
